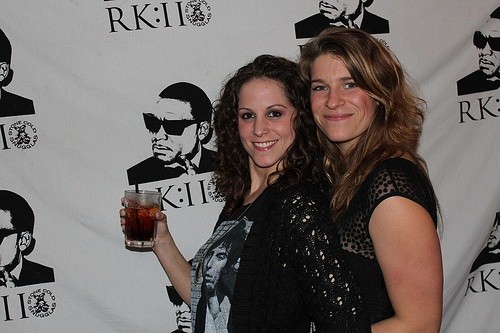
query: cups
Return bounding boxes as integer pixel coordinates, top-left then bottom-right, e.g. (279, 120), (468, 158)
(125, 190), (162, 252)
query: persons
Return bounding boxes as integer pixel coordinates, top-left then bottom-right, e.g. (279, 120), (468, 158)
(298, 26), (444, 333)
(120, 54), (375, 333)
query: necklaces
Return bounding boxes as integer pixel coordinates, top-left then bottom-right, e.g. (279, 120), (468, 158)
(194, 202), (252, 286)
